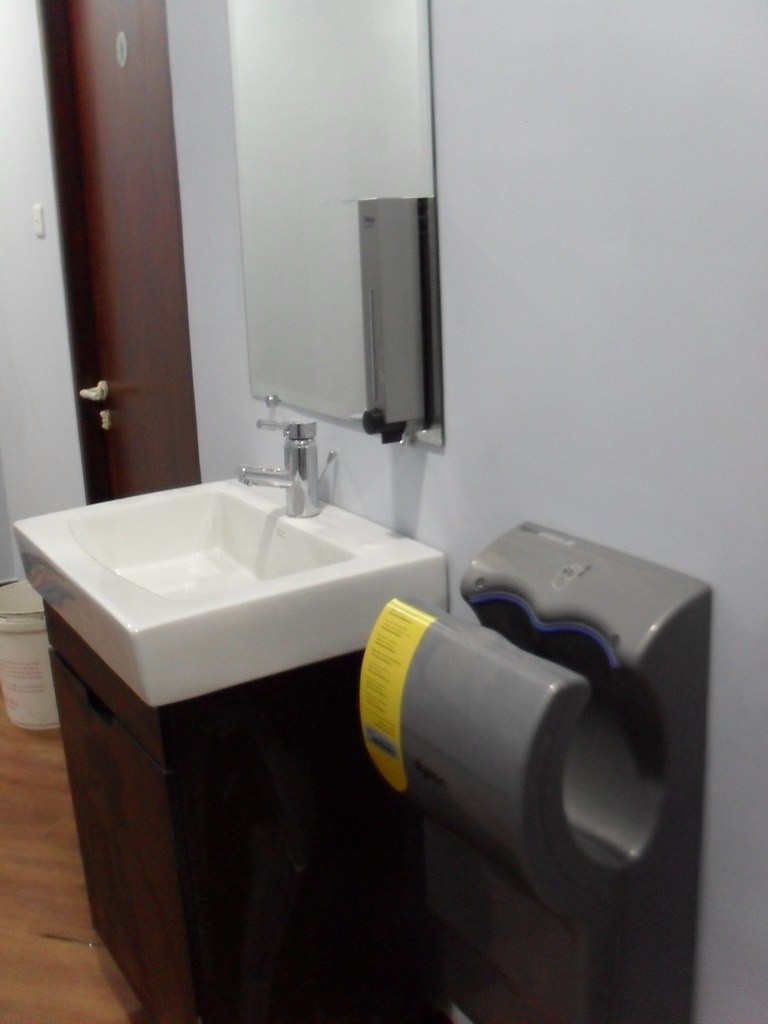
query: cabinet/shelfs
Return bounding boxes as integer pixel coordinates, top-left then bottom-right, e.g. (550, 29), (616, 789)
(47, 648), (440, 1024)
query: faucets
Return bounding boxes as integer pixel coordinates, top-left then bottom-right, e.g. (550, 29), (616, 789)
(238, 419), (338, 517)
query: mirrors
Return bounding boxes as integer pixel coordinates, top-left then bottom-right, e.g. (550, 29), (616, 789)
(227, 0), (443, 453)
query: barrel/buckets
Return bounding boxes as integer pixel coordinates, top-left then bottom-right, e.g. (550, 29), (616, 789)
(0, 579), (61, 731)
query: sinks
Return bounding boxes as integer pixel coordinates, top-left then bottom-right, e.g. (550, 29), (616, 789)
(12, 475), (447, 708)
